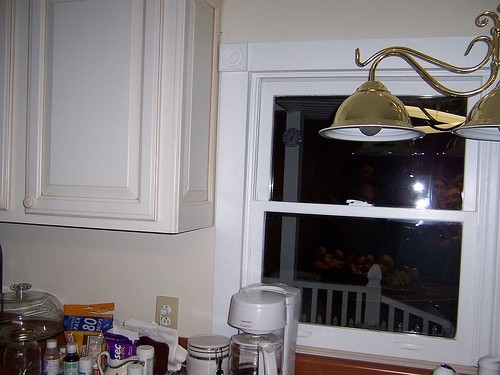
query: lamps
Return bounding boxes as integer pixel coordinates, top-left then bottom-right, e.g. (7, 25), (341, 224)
(319, 0), (500, 141)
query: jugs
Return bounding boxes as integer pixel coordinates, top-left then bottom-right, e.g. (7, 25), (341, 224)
(230, 333), (283, 375)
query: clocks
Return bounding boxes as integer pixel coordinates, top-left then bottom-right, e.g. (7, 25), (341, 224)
(283, 128), (302, 147)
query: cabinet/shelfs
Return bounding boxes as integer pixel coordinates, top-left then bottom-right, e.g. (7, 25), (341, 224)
(0, 0), (219, 234)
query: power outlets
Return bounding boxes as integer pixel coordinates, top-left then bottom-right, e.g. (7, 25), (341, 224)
(154, 296), (178, 329)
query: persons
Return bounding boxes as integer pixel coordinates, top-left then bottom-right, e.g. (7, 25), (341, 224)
(306, 173), (464, 289)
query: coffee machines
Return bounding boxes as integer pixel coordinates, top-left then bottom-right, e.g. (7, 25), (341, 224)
(228, 282), (302, 375)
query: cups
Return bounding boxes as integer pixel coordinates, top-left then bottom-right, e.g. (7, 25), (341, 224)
(97, 351), (138, 375)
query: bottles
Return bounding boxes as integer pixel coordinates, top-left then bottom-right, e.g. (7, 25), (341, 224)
(187, 334), (230, 375)
(3, 327), (42, 375)
(478, 354), (500, 375)
(127, 345), (154, 375)
(43, 338), (92, 375)
